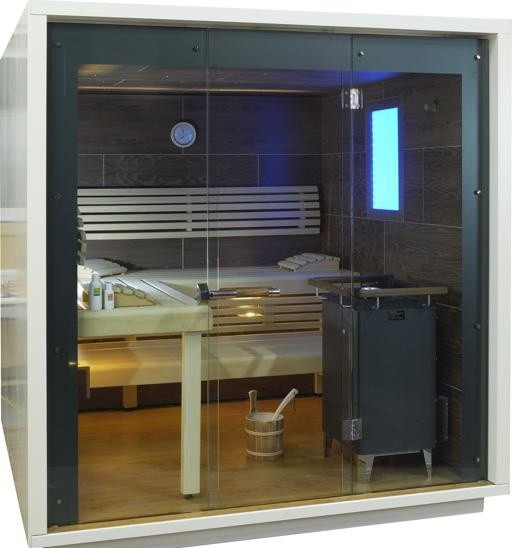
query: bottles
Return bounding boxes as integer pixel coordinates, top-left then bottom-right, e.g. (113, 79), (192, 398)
(104, 282), (114, 309)
(89, 274), (102, 310)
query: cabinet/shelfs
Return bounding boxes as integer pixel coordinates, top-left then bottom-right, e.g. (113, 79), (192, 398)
(306, 276), (450, 484)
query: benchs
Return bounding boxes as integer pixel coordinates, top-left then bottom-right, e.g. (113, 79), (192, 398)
(74, 186), (362, 296)
(78, 289), (323, 389)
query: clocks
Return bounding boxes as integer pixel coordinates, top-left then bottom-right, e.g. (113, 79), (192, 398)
(170, 121), (197, 148)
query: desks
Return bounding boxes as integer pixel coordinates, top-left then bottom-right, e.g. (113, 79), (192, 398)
(77, 207), (214, 500)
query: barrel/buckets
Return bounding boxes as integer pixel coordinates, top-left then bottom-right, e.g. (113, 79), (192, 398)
(244, 389), (286, 463)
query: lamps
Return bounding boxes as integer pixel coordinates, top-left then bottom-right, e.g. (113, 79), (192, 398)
(339, 38), (365, 114)
